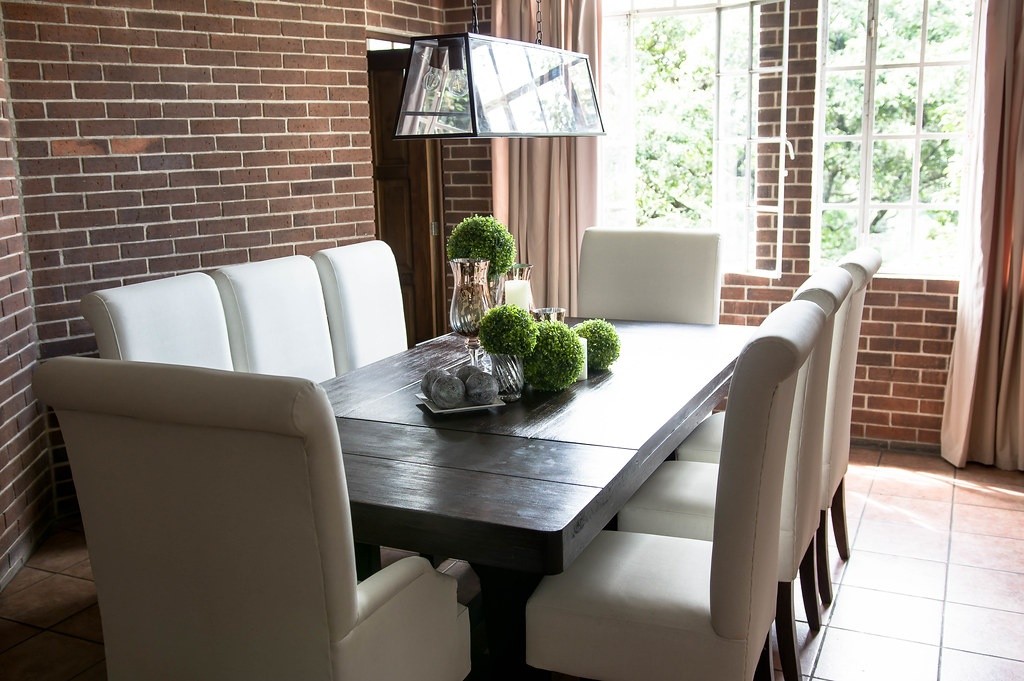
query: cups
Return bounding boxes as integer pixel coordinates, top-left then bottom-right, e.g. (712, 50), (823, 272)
(495, 264), (534, 307)
(531, 308), (566, 328)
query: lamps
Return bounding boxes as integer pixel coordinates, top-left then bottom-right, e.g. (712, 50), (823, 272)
(394, 0), (606, 140)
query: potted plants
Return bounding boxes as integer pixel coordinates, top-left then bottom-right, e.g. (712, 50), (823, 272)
(479, 310), (623, 400)
(446, 217), (516, 308)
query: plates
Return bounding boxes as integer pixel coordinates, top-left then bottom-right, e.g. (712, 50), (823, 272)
(416, 392), (506, 415)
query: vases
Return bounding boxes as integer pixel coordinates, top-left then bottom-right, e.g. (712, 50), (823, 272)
(449, 259), (490, 336)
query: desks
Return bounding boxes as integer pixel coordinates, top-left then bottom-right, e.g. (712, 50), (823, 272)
(317, 319), (764, 681)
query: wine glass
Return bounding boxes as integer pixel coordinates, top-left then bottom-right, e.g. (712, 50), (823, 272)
(449, 258), (493, 367)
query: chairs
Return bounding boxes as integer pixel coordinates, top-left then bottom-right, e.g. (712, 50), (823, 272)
(81, 273), (233, 382)
(313, 241), (409, 376)
(676, 248), (882, 610)
(214, 256), (336, 398)
(615, 267), (852, 681)
(32, 358), (473, 681)
(526, 300), (825, 681)
(577, 227), (722, 326)
